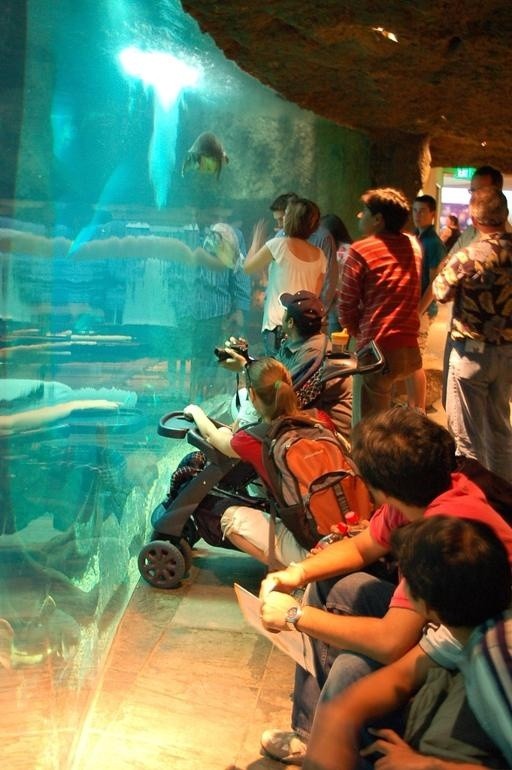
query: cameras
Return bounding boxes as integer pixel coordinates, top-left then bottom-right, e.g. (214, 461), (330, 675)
(214, 341), (251, 365)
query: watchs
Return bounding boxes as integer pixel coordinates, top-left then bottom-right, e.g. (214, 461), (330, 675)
(285, 605), (302, 632)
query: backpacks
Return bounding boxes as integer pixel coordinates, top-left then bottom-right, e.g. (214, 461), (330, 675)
(246, 407), (376, 551)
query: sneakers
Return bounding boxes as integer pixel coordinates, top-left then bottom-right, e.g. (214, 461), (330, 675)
(262, 729), (309, 767)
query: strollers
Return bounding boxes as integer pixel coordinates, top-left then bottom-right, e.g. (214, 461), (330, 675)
(137, 336), (387, 590)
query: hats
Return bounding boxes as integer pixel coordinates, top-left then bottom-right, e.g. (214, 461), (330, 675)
(281, 291), (326, 330)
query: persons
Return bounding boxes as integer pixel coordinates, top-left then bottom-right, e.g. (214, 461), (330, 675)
(252, 403), (512, 769)
(305, 514), (512, 769)
(185, 162), (512, 578)
